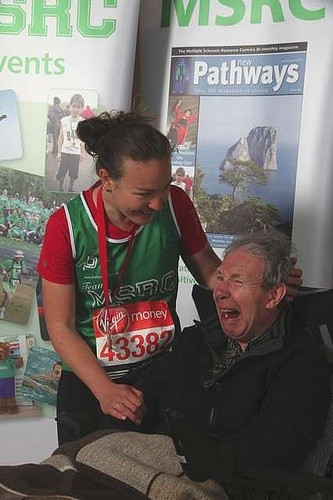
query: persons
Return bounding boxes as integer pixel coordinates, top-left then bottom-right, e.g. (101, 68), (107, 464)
(0, 94), (97, 319)
(24, 361), (66, 406)
(73, 228), (333, 483)
(170, 168), (192, 197)
(35, 111), (304, 446)
(168, 100), (200, 146)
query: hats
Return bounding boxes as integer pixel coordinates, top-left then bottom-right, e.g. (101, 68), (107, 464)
(14, 251), (24, 259)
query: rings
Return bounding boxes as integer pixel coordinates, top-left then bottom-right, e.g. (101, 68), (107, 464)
(114, 401), (121, 409)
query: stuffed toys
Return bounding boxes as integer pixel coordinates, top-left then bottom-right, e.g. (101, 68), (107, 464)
(0, 342), (25, 415)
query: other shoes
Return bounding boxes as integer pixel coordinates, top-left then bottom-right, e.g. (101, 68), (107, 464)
(68, 186), (75, 191)
(0, 307), (5, 319)
(59, 187), (64, 191)
